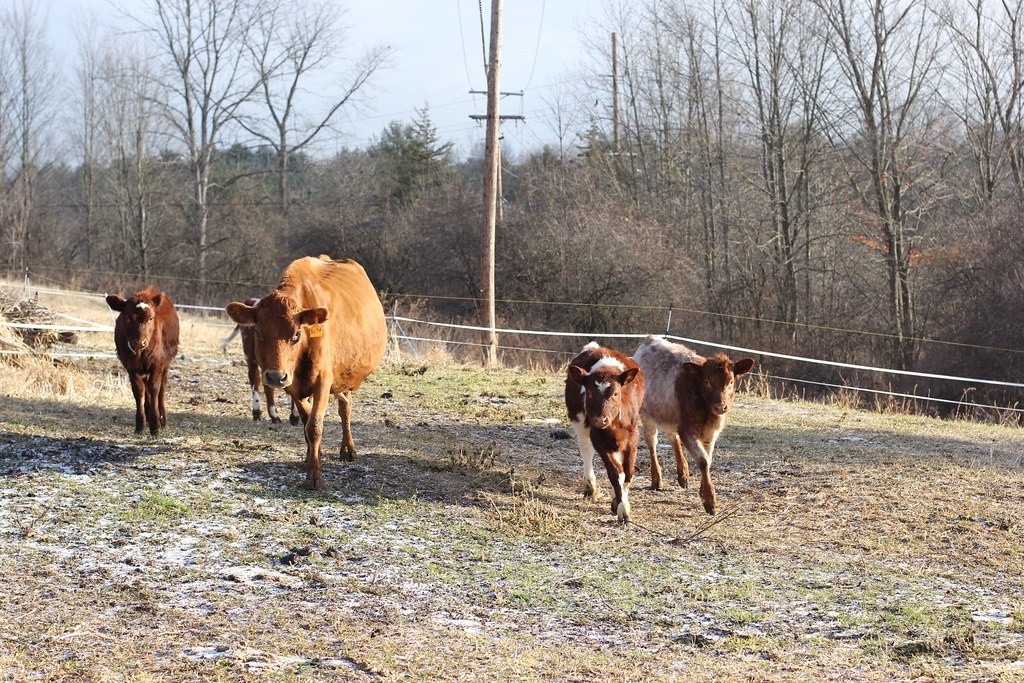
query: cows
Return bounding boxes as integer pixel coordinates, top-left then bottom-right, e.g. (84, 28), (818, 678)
(226, 253), (389, 488)
(565, 341), (647, 524)
(104, 284), (180, 440)
(634, 335), (755, 514)
